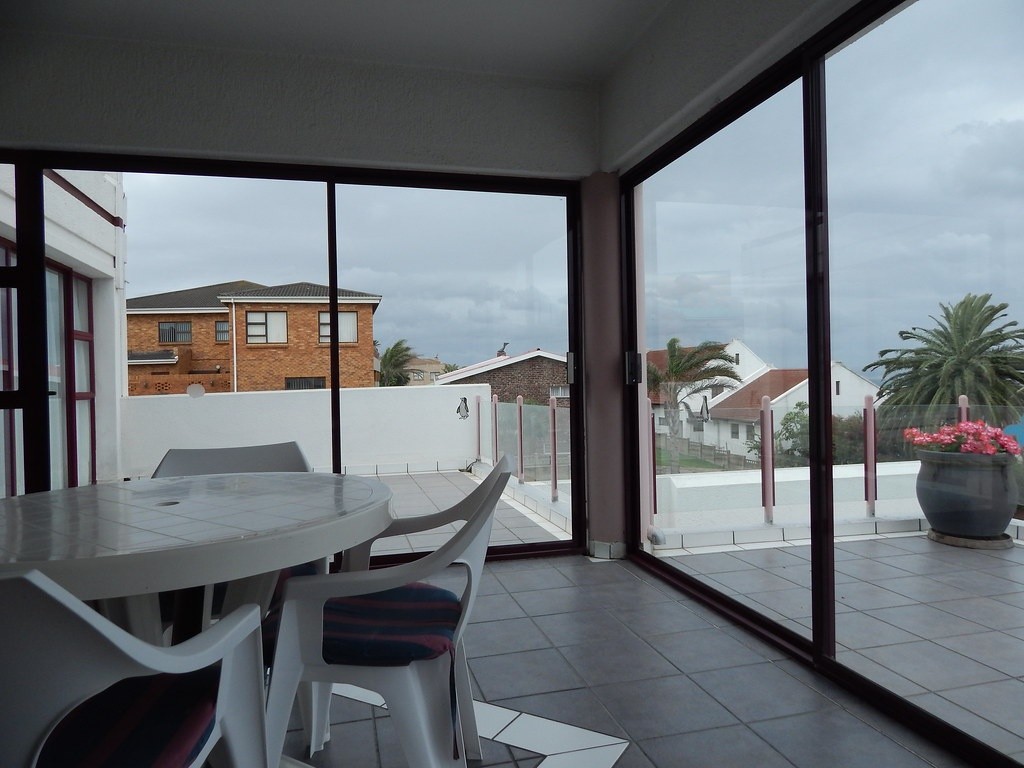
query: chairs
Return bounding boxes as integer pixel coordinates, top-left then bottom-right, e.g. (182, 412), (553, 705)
(266, 451), (513, 768)
(152, 441), (331, 747)
(0, 568), (268, 768)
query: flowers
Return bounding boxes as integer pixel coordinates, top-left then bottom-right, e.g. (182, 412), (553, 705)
(903, 419), (1024, 458)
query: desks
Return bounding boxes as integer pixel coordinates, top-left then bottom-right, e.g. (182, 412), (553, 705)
(0, 471), (395, 768)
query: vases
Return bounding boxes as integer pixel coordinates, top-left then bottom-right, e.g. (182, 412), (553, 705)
(914, 448), (1020, 539)
